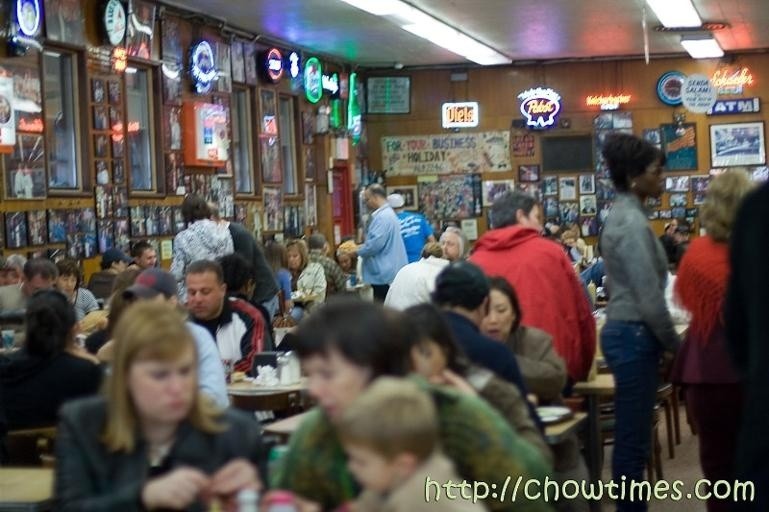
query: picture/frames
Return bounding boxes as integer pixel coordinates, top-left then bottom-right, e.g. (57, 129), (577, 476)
(365, 73), (634, 238)
(0, 10), (318, 266)
(642, 120), (765, 220)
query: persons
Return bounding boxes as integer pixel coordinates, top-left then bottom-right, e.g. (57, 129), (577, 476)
(0, 132), (769, 512)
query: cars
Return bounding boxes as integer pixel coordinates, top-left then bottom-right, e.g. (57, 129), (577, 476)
(1, 329), (16, 353)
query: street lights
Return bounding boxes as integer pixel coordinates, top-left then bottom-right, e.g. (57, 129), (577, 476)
(271, 315), (297, 327)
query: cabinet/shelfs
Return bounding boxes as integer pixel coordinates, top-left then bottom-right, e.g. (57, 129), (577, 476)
(350, 271), (357, 286)
(267, 491), (296, 512)
(236, 485), (258, 512)
(587, 279), (597, 307)
(276, 356), (290, 384)
(285, 349), (301, 382)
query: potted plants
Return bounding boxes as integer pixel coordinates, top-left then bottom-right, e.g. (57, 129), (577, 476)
(642, 120), (765, 220)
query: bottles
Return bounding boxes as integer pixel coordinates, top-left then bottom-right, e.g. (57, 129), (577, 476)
(121, 267), (177, 300)
(101, 248), (132, 264)
(387, 193), (405, 209)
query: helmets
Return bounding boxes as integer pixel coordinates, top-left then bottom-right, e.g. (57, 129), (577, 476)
(536, 406), (571, 421)
